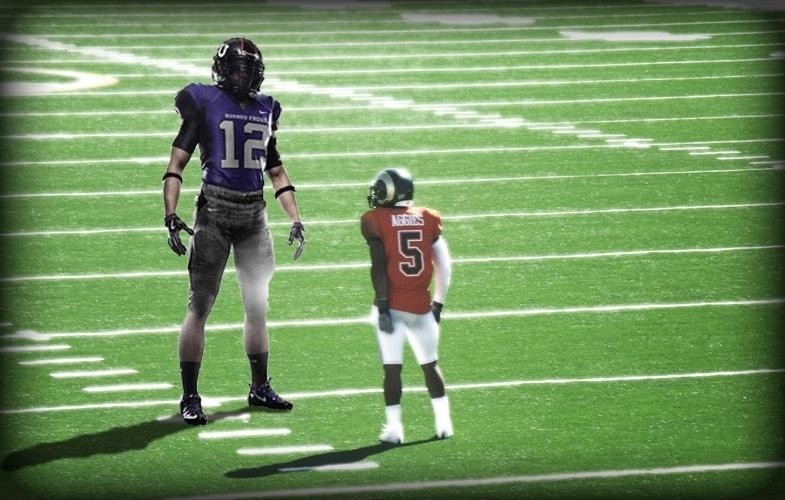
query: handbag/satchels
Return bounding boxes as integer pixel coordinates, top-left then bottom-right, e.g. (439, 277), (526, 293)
(375, 295), (389, 309)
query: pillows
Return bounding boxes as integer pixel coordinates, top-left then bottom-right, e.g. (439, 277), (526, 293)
(366, 167), (416, 209)
(210, 37), (267, 94)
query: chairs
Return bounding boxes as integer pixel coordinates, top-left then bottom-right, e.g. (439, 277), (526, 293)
(379, 421), (407, 448)
(247, 379), (294, 414)
(178, 392), (210, 429)
(433, 418), (456, 441)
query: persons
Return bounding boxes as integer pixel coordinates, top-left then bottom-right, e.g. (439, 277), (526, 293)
(359, 165), (454, 447)
(161, 36), (308, 427)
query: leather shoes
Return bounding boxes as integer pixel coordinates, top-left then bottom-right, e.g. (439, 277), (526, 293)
(286, 222), (309, 260)
(374, 296), (398, 335)
(162, 212), (195, 257)
(429, 300), (444, 324)
